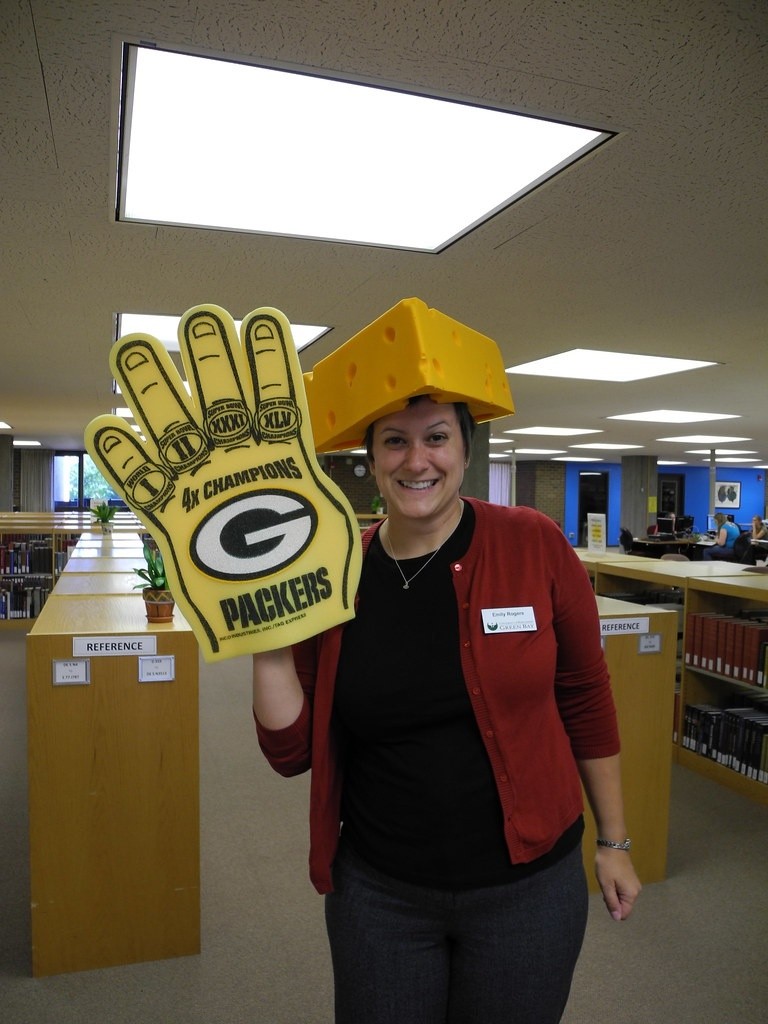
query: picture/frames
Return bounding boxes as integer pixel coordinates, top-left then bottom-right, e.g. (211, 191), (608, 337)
(715, 481), (741, 508)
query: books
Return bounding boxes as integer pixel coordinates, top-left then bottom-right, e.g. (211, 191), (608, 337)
(682, 701), (768, 786)
(0, 534), (78, 619)
(684, 609), (767, 688)
(596, 588), (684, 606)
(672, 687), (679, 743)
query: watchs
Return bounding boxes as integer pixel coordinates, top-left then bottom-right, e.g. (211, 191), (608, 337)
(591, 836), (634, 853)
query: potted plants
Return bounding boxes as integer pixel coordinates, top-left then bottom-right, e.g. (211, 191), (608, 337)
(88, 500), (118, 534)
(133, 542), (175, 622)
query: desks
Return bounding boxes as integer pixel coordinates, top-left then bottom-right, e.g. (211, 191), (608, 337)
(632, 534), (716, 560)
(750, 538), (768, 542)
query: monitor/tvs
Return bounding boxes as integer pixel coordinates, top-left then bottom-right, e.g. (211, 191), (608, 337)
(674, 516), (694, 532)
(658, 518), (674, 533)
(707, 515), (728, 532)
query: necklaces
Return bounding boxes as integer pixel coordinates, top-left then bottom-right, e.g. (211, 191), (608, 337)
(382, 498), (466, 591)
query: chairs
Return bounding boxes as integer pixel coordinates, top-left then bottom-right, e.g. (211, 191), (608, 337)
(660, 553), (690, 561)
(711, 531), (754, 564)
(620, 527), (647, 557)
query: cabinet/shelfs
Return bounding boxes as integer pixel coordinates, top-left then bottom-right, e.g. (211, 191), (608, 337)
(596, 560), (765, 764)
(575, 550), (673, 594)
(555, 594), (678, 895)
(677, 574), (768, 811)
(0, 504), (201, 977)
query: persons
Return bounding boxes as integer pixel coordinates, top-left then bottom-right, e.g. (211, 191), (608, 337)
(739, 515), (768, 563)
(663, 511), (678, 532)
(247, 393), (645, 1024)
(701, 512), (740, 560)
